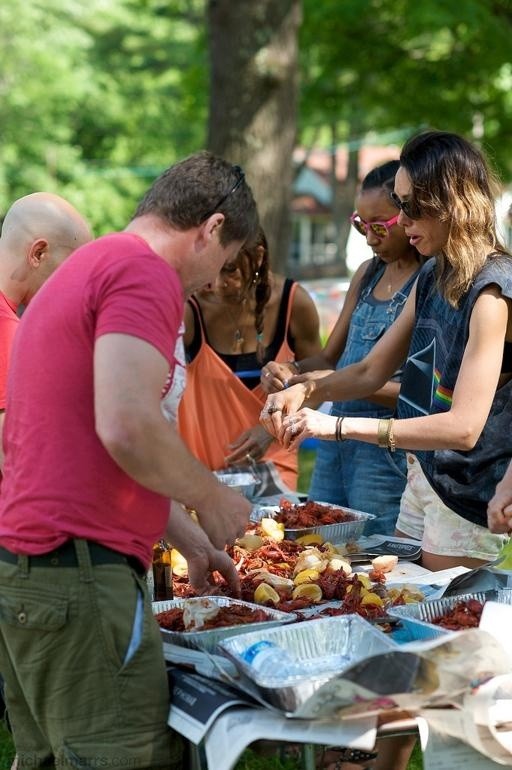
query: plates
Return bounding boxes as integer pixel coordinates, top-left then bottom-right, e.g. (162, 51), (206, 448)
(219, 613), (409, 708)
(153, 593), (300, 652)
(390, 589), (512, 640)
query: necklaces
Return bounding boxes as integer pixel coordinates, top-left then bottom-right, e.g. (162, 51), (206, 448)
(387, 263), (416, 293)
(223, 301), (251, 354)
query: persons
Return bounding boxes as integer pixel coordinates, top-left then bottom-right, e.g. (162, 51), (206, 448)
(0, 152), (257, 770)
(264, 161), (431, 769)
(487, 458), (512, 534)
(259, 132), (512, 769)
(0, 192), (94, 476)
(175, 228), (323, 492)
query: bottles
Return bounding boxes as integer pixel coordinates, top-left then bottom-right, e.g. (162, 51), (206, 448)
(152, 548), (173, 601)
(235, 638), (307, 688)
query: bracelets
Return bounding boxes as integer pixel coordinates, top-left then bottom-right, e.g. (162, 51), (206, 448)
(378, 418), (388, 448)
(291, 362), (303, 374)
(389, 418), (396, 452)
(335, 416), (345, 441)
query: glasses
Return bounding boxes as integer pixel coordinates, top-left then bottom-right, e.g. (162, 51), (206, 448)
(390, 191), (422, 220)
(351, 212), (399, 241)
(200, 165), (245, 223)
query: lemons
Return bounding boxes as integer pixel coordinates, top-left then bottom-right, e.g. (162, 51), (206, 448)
(170, 517), (419, 609)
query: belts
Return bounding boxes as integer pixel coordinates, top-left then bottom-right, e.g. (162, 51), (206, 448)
(1, 541), (127, 568)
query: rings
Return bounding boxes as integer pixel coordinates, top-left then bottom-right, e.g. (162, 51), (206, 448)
(291, 425), (298, 435)
(265, 372), (271, 377)
(246, 454), (253, 462)
(289, 417), (297, 424)
(268, 405), (282, 413)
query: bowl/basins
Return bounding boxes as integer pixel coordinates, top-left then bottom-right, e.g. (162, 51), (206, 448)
(250, 502), (376, 545)
(213, 471), (258, 504)
